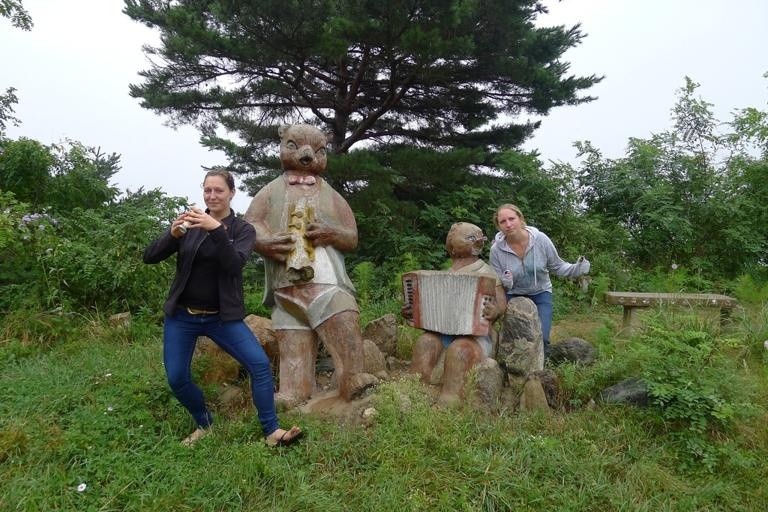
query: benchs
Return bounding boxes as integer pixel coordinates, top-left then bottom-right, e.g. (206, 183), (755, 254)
(604, 291), (737, 335)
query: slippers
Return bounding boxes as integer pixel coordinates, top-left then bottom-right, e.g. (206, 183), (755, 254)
(265, 431), (304, 448)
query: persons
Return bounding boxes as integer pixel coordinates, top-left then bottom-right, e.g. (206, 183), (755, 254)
(143, 168), (302, 446)
(489, 203), (591, 359)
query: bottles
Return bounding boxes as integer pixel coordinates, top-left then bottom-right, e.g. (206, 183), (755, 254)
(178, 210), (197, 233)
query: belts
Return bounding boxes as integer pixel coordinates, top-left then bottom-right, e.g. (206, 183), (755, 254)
(176, 302), (220, 316)
